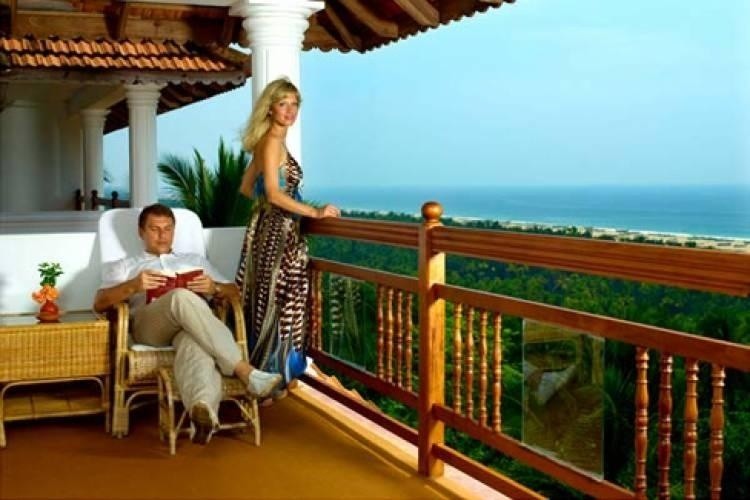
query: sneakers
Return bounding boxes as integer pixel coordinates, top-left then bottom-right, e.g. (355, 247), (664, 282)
(246, 368), (283, 400)
(188, 400), (218, 445)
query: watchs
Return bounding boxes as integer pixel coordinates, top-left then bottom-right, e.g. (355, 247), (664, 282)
(214, 283), (224, 300)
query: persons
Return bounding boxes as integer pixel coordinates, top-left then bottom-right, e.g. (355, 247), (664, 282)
(94, 204), (282, 445)
(226, 80), (340, 407)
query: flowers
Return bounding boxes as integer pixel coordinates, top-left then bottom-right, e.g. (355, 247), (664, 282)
(31, 262), (64, 303)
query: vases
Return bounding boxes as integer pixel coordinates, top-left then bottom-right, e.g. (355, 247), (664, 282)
(37, 298), (61, 322)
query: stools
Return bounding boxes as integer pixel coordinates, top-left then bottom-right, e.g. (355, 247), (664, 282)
(156, 363), (262, 456)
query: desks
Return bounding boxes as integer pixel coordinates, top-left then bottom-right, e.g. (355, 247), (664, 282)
(0, 309), (113, 450)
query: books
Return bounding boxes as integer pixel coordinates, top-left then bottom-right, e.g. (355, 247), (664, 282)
(145, 267), (205, 305)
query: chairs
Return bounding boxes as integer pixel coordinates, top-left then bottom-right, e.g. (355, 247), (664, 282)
(98, 207), (251, 439)
